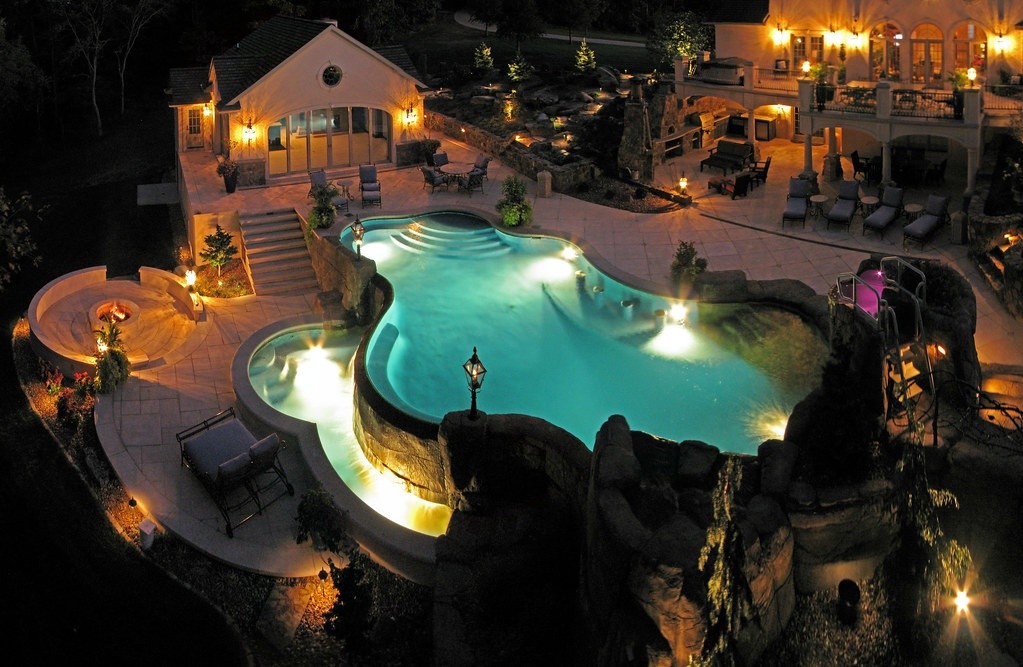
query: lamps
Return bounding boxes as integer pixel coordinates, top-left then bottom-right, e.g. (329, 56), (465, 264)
(462, 346), (487, 423)
(679, 170), (689, 194)
(405, 103), (416, 125)
(351, 214), (367, 264)
(184, 264), (198, 296)
(243, 117), (255, 145)
(204, 104), (210, 116)
(802, 58), (811, 77)
(967, 64), (976, 87)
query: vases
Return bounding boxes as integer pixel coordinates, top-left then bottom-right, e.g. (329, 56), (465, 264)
(223, 170), (237, 194)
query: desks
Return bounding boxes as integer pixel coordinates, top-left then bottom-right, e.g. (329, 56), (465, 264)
(904, 203), (922, 222)
(810, 195), (829, 220)
(741, 170), (760, 190)
(700, 157), (740, 177)
(440, 163), (475, 192)
(866, 155), (925, 188)
(336, 179), (354, 201)
(861, 196), (879, 215)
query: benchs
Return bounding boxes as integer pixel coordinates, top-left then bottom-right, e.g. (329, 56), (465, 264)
(706, 140), (752, 170)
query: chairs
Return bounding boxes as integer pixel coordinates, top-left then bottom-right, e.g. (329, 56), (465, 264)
(861, 186), (905, 241)
(305, 167), (336, 200)
(826, 179), (862, 232)
(923, 157), (948, 188)
(433, 151), (457, 181)
(772, 58), (790, 79)
(851, 150), (870, 180)
(175, 406), (296, 541)
(894, 146), (908, 159)
(358, 163), (378, 192)
(467, 153), (493, 181)
(903, 193), (951, 253)
(748, 156), (773, 183)
(360, 179), (382, 209)
(911, 147), (925, 161)
(421, 167), (450, 194)
(457, 170), (486, 198)
(708, 172), (752, 200)
(782, 176), (810, 230)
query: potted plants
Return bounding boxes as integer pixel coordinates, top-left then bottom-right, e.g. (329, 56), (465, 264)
(837, 65), (847, 84)
(948, 65), (967, 117)
(932, 73), (943, 83)
(811, 63), (834, 101)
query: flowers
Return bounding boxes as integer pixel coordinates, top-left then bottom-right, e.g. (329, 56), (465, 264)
(217, 159), (240, 176)
